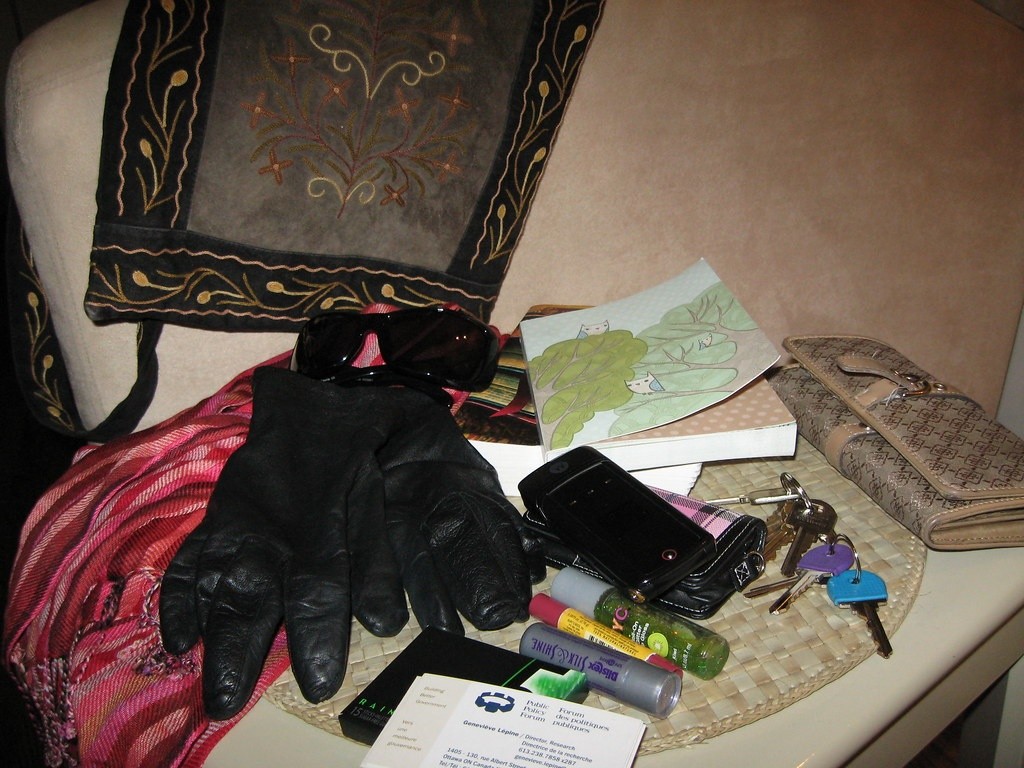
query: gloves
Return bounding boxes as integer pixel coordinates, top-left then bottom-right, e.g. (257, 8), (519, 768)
(159, 364), (405, 723)
(349, 383), (549, 644)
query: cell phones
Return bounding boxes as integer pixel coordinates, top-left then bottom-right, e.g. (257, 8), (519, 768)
(519, 446), (717, 605)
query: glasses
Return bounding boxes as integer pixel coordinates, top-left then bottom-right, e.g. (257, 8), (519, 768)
(288, 302), (502, 399)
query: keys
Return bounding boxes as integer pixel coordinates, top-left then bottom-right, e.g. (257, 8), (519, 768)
(703, 471), (894, 660)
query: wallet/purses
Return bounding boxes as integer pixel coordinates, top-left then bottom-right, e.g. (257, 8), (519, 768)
(518, 482), (769, 623)
(766, 332), (1023, 552)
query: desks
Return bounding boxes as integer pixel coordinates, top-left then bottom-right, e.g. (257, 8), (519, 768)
(84, 437), (1024, 768)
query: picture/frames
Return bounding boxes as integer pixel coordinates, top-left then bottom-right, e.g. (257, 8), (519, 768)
(86, 0), (607, 337)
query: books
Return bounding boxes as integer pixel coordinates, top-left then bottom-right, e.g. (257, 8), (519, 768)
(520, 257), (797, 473)
